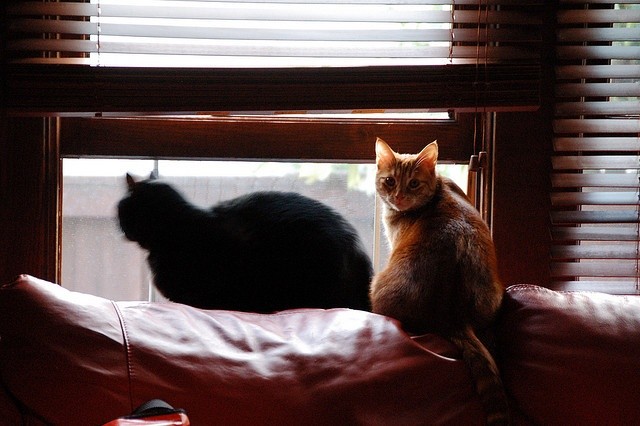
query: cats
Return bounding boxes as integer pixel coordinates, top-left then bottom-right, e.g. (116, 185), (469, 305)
(117, 170), (371, 313)
(368, 135), (513, 426)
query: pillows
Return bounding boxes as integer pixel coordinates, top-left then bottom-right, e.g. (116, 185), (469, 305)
(482, 280), (639, 426)
(1, 271), (526, 425)
